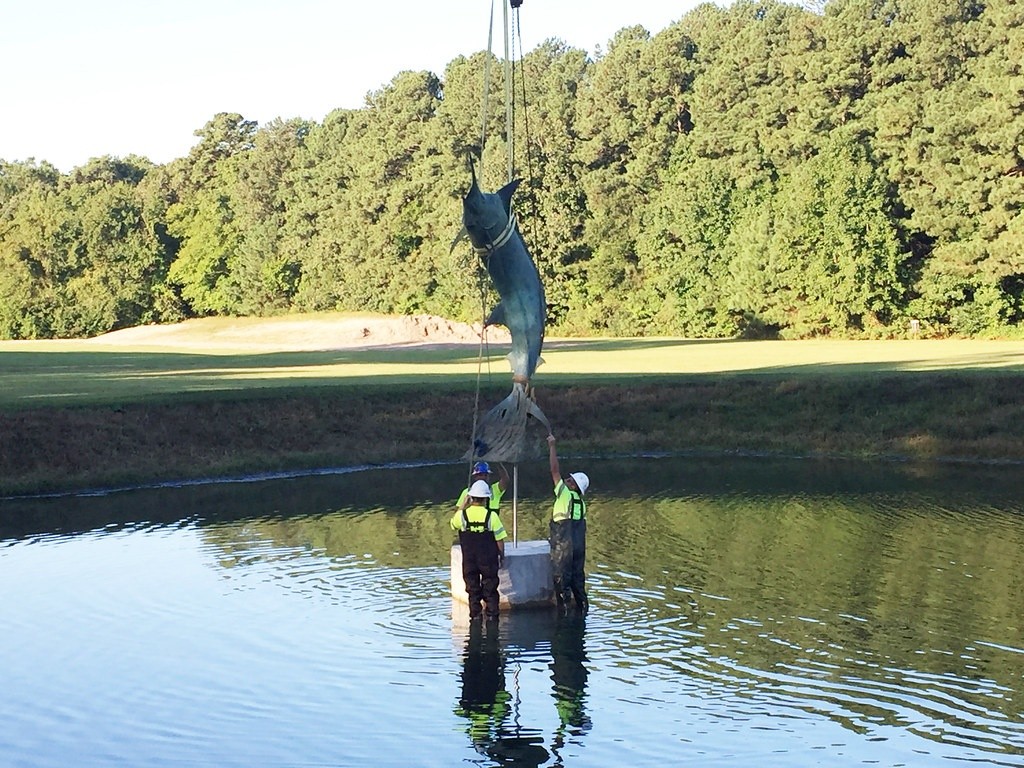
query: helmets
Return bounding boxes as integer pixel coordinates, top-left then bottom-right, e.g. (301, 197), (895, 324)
(467, 480), (492, 497)
(570, 472), (589, 495)
(471, 462), (493, 475)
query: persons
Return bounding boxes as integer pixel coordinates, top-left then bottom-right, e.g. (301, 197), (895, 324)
(450, 480), (508, 619)
(545, 433), (589, 616)
(455, 461), (510, 515)
